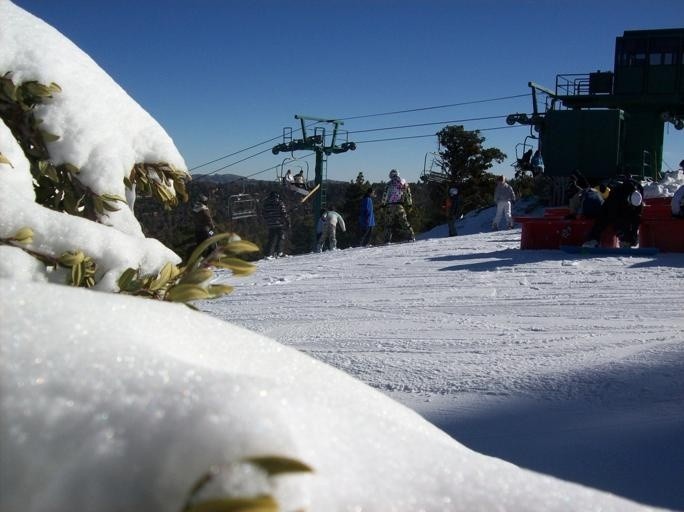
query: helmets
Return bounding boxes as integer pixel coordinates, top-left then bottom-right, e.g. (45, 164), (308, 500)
(197, 192), (208, 205)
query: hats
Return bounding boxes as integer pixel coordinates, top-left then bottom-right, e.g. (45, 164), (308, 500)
(448, 187), (458, 195)
(319, 208), (328, 217)
(389, 168), (400, 178)
(627, 190), (643, 207)
(270, 191), (279, 198)
(329, 206), (337, 211)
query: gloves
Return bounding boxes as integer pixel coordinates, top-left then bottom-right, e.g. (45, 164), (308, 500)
(408, 206), (412, 214)
(380, 208), (384, 214)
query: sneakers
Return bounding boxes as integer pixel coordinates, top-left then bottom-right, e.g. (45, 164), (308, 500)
(619, 241), (630, 249)
(582, 240), (601, 249)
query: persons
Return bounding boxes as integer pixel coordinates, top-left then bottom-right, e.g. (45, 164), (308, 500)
(381, 169), (416, 245)
(293, 170), (306, 190)
(191, 195), (217, 259)
(261, 191), (293, 259)
(670, 160), (684, 219)
(564, 172), (644, 249)
(352, 187), (376, 248)
(284, 169), (294, 183)
(312, 208), (326, 253)
(491, 176), (516, 230)
(316, 205), (346, 253)
(447, 187), (464, 237)
